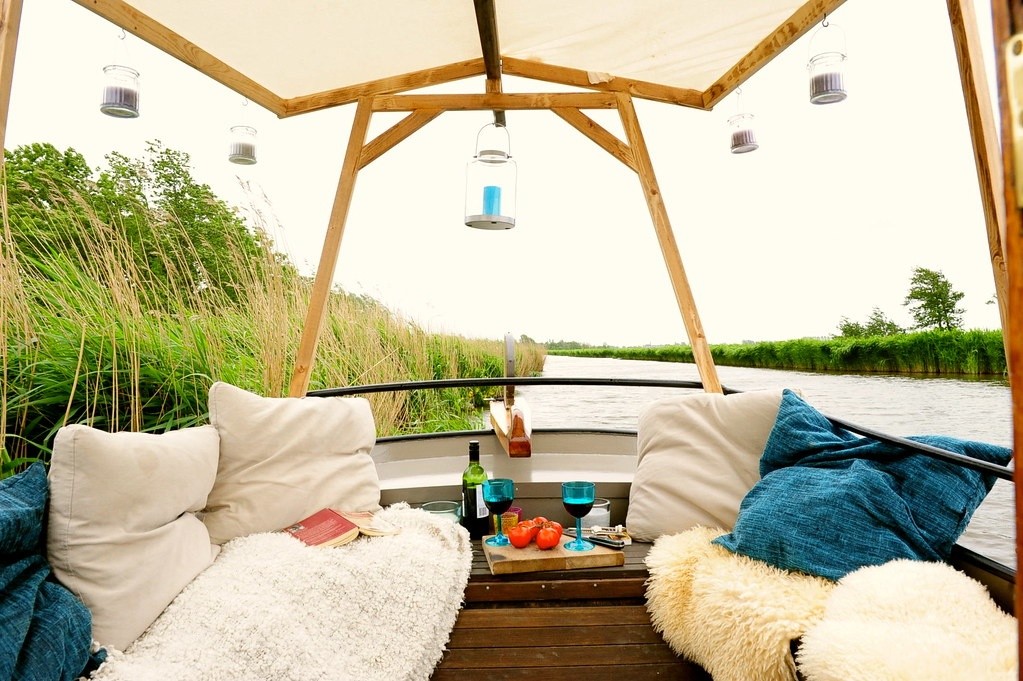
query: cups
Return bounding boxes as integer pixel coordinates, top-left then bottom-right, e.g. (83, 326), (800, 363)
(581, 497), (610, 530)
(422, 500), (459, 514)
(503, 506), (522, 523)
(493, 512), (518, 535)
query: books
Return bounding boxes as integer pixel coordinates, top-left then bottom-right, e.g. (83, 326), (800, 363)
(282, 507), (403, 549)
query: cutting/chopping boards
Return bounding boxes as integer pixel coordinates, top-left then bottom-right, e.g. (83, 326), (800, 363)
(482, 531), (625, 575)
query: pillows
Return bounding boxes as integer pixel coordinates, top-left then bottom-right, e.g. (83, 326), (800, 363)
(47, 424), (222, 652)
(0, 462), (108, 681)
(711, 389), (1015, 584)
(195, 382), (381, 546)
(626, 389), (803, 541)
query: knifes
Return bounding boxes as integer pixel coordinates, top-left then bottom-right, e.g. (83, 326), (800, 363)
(563, 532), (624, 550)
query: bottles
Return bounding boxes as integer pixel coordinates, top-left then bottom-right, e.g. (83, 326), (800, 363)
(460, 440), (490, 540)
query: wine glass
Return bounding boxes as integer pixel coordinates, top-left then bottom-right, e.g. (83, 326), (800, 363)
(561, 481), (595, 551)
(481, 478), (514, 547)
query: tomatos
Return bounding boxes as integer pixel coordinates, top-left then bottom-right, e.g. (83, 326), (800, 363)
(508, 516), (562, 550)
